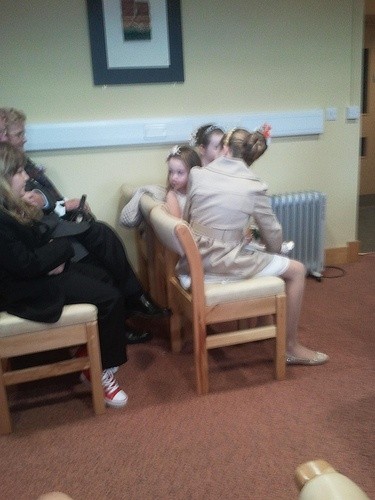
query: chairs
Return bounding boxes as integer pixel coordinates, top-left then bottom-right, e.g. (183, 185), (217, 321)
(0, 303), (106, 436)
(118, 182), (287, 395)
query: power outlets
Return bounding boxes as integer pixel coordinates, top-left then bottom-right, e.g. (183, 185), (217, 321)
(325, 107), (337, 121)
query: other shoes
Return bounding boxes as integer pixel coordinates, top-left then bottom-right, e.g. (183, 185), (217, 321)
(286, 351), (328, 365)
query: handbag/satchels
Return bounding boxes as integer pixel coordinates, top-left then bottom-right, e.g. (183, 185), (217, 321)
(66, 195), (95, 246)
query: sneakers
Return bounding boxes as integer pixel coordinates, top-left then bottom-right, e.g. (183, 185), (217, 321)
(80, 368), (128, 405)
(74, 366), (119, 395)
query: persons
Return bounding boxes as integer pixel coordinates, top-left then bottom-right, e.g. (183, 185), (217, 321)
(0, 109), (292, 408)
(184, 127), (330, 365)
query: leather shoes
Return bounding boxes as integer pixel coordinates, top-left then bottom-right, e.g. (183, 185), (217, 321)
(123, 329), (152, 343)
(127, 292), (171, 319)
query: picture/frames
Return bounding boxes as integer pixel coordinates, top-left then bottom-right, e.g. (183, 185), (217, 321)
(85, 0), (184, 86)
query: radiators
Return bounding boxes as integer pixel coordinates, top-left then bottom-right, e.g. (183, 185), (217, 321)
(270, 190), (327, 283)
